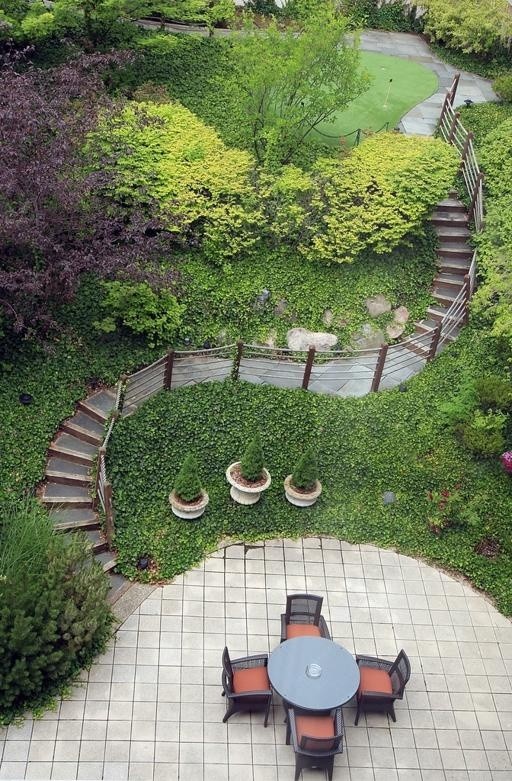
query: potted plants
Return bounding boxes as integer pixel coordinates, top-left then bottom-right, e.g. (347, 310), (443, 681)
(226, 431), (271, 505)
(284, 447), (322, 506)
(169, 455), (210, 519)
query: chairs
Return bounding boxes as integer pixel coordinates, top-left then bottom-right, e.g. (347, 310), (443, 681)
(280, 594), (331, 643)
(354, 649), (410, 726)
(286, 706), (344, 781)
(222, 646), (273, 727)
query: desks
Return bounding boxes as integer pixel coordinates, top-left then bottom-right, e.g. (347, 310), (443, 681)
(267, 635), (361, 723)
